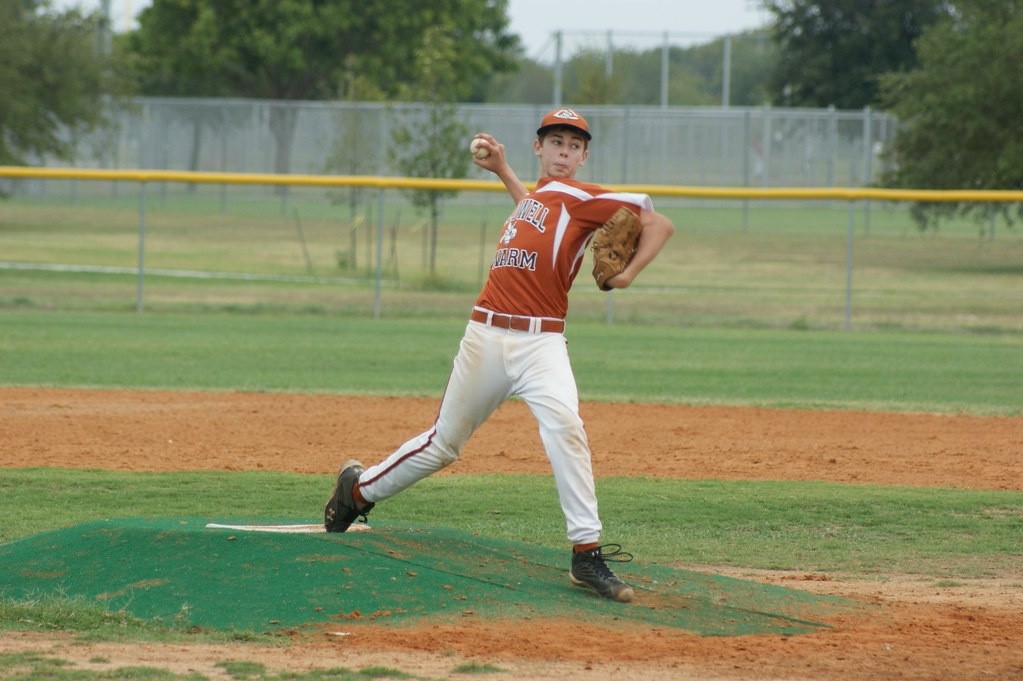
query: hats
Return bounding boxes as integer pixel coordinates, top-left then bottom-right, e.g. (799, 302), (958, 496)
(536, 108), (592, 140)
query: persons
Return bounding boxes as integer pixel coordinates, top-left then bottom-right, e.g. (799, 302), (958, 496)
(322, 109), (674, 603)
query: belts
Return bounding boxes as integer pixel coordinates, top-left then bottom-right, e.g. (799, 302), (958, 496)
(471, 310), (564, 333)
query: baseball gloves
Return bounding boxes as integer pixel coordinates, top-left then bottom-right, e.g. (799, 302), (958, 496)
(589, 204), (644, 292)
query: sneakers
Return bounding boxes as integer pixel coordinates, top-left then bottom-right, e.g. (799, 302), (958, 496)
(323, 458), (375, 532)
(570, 544), (635, 601)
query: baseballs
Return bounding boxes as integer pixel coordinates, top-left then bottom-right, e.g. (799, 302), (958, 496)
(471, 137), (490, 159)
